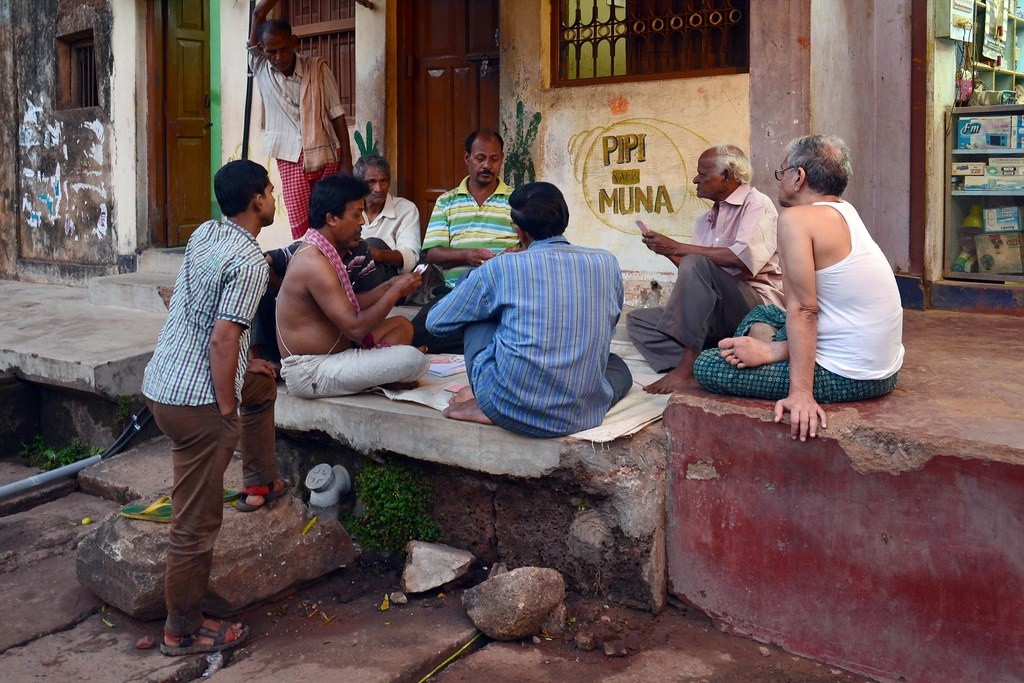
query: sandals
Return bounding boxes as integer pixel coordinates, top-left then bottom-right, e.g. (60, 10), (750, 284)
(235, 477), (289, 511)
(160, 619), (250, 655)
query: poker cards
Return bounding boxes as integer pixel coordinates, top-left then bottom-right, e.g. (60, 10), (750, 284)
(347, 254), (377, 278)
(431, 355), (465, 364)
(635, 220), (651, 236)
(479, 247), (494, 260)
(413, 263), (429, 275)
(443, 383), (466, 392)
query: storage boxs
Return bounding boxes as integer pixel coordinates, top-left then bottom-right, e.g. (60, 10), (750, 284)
(952, 116), (1024, 275)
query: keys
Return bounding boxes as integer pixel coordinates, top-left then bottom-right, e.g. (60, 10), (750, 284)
(312, 383), (317, 394)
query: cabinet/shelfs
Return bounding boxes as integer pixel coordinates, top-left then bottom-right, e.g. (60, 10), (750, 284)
(973, 0), (1024, 105)
(943, 102), (1024, 282)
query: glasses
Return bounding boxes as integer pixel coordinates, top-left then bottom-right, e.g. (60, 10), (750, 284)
(775, 166), (798, 180)
(511, 221), (517, 230)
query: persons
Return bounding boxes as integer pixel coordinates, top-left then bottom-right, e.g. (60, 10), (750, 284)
(139, 159), (294, 658)
(276, 175), (430, 399)
(421, 130), (633, 438)
(627, 135), (786, 393)
(692, 133), (905, 442)
(353, 155), (421, 305)
(246, 0), (353, 242)
